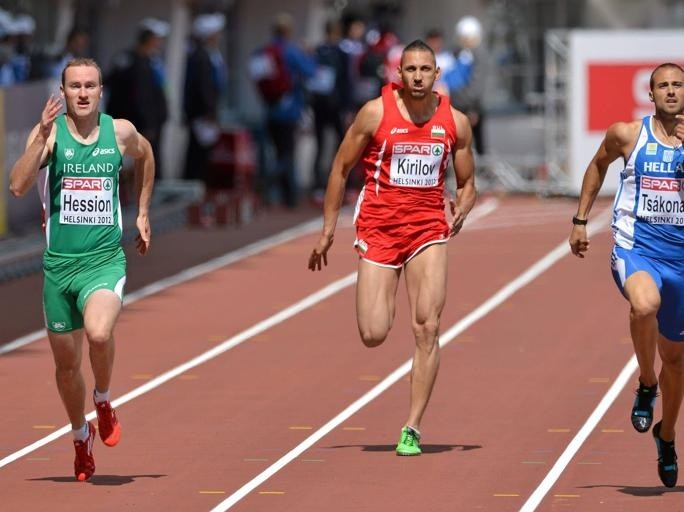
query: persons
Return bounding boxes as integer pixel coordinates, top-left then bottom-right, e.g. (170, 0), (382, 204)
(7, 58), (157, 482)
(100, 12), (496, 230)
(1, 0), (89, 85)
(308, 39), (474, 457)
(569, 63), (684, 488)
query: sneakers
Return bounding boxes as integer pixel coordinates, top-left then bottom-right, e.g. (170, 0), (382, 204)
(653, 420), (678, 487)
(396, 424), (422, 455)
(93, 392), (121, 445)
(631, 377), (658, 433)
(72, 421), (96, 480)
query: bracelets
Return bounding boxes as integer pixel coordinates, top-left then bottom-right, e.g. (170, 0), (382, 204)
(571, 217), (588, 225)
